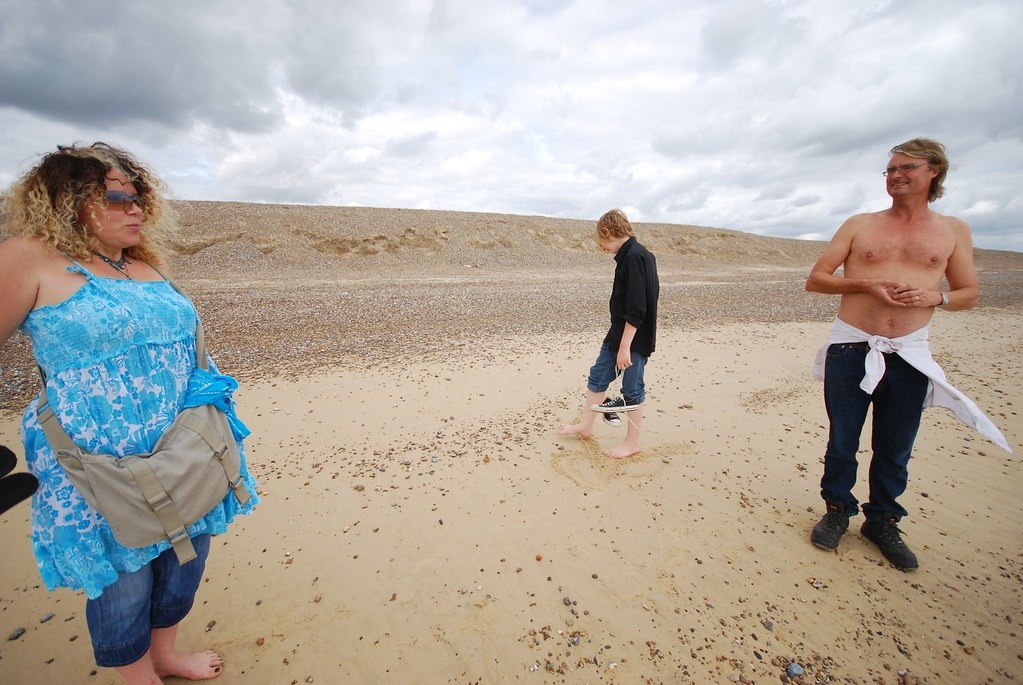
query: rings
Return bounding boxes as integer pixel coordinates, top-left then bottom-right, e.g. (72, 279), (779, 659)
(915, 296), (918, 301)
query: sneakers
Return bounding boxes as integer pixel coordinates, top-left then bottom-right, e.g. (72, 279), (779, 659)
(860, 511), (918, 570)
(591, 364), (645, 431)
(811, 500), (849, 551)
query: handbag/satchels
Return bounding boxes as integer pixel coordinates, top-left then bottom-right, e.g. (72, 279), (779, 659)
(56, 404), (253, 567)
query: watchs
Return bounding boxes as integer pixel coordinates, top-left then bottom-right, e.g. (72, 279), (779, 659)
(934, 290), (948, 308)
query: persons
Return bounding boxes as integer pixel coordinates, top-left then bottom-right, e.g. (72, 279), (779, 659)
(556, 208), (660, 460)
(0, 139), (261, 685)
(805, 138), (979, 572)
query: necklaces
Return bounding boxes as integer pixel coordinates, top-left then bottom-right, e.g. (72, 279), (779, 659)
(86, 248), (131, 281)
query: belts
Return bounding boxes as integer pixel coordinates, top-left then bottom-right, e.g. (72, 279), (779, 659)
(832, 342), (894, 358)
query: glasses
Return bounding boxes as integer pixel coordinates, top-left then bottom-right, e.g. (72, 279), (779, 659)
(883, 162), (933, 177)
(86, 190), (145, 211)
